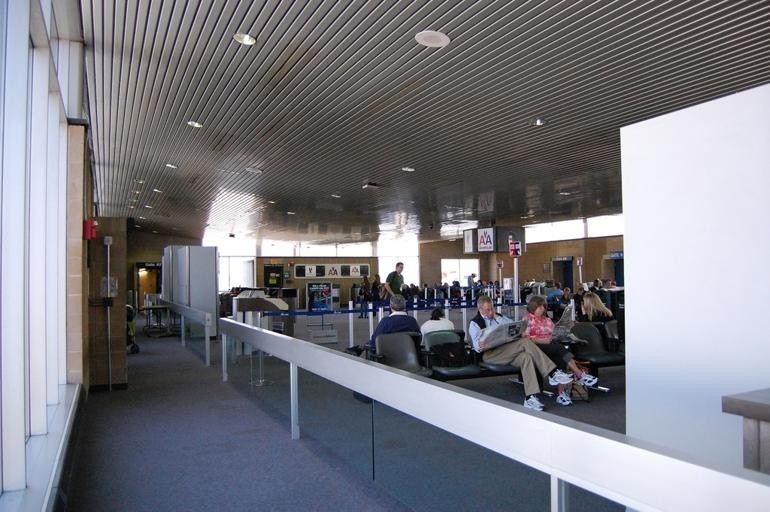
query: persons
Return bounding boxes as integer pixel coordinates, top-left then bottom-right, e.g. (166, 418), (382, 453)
(353, 262), (624, 321)
(520, 296), (599, 407)
(343, 293), (422, 407)
(418, 308), (455, 347)
(468, 295), (574, 412)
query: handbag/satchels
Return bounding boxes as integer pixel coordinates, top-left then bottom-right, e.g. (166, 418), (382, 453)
(565, 380), (589, 401)
(430, 343), (471, 368)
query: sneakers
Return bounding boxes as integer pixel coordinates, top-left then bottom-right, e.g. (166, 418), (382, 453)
(524, 395), (543, 411)
(557, 393), (572, 406)
(575, 372), (598, 386)
(344, 346), (362, 356)
(549, 369), (573, 386)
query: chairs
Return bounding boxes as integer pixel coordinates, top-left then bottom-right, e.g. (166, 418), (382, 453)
(373, 319), (625, 403)
